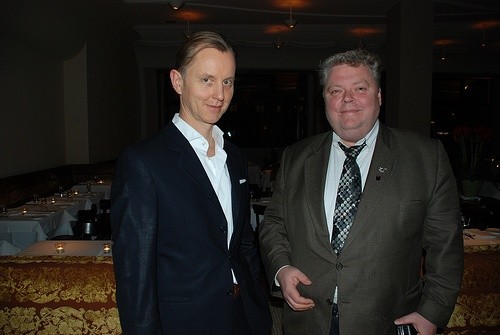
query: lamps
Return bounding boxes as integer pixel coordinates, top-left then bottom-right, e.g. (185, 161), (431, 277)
(168, 0), (186, 11)
(183, 15), (194, 39)
(273, 32), (285, 49)
(283, 7), (298, 30)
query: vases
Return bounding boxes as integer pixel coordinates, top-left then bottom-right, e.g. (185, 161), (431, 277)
(461, 179), (484, 198)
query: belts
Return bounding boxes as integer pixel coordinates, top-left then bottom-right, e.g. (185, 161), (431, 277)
(232, 285), (240, 298)
(332, 308), (339, 318)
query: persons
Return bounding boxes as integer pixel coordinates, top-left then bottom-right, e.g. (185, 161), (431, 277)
(111, 30), (273, 334)
(258, 47), (464, 334)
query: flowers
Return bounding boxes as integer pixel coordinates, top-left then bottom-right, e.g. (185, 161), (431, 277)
(453, 124), (495, 181)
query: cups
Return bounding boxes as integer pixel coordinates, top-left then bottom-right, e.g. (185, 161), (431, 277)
(58, 183), (64, 194)
(104, 243), (110, 253)
(55, 243), (63, 254)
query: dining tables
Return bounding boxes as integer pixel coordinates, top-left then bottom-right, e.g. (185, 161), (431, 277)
(0, 180), (500, 335)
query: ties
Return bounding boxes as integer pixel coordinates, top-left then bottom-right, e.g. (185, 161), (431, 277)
(331, 141), (367, 255)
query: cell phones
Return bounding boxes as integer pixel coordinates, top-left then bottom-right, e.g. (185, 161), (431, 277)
(398, 324), (418, 335)
